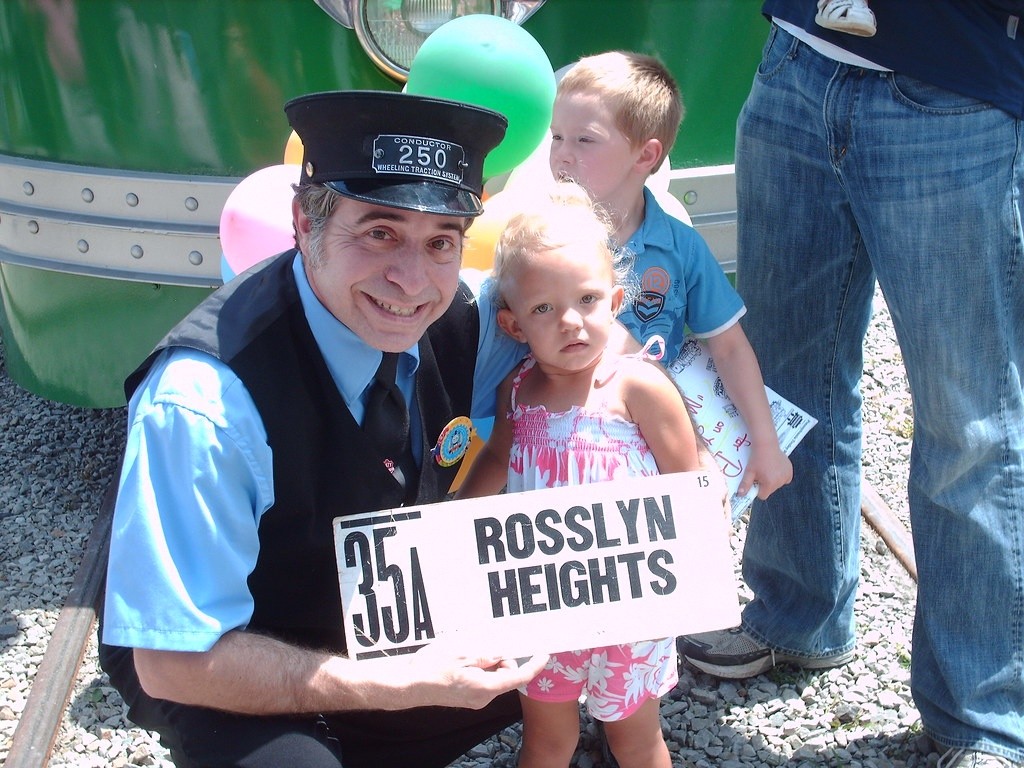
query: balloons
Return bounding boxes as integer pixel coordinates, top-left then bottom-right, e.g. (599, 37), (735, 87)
(217, 12), (694, 285)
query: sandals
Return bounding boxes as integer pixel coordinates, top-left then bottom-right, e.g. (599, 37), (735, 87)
(815, 0), (877, 37)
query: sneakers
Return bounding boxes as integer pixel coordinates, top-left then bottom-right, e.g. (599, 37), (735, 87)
(676, 627), (855, 679)
(933, 740), (1024, 768)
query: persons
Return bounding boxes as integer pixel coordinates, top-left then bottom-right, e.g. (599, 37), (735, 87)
(1, 1), (385, 184)
(452, 200), (703, 767)
(669, 1), (1024, 767)
(92, 90), (526, 767)
(549, 47), (795, 503)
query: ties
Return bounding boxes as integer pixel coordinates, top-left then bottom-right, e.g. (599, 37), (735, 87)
(362, 352), (419, 505)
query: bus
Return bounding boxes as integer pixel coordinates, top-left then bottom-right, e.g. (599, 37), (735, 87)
(0, 0), (778, 410)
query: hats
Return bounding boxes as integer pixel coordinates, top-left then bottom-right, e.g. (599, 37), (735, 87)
(283, 89), (508, 217)
